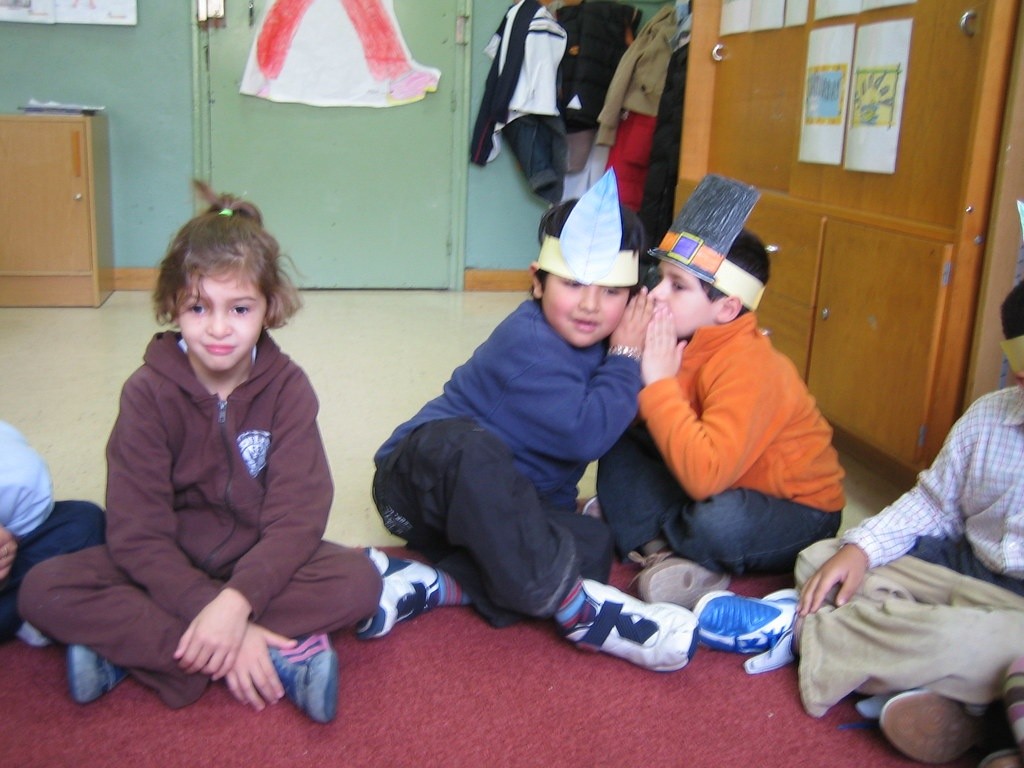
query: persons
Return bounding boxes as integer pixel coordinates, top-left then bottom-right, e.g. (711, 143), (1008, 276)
(355, 199), (699, 671)
(693, 279), (1024, 768)
(17, 180), (384, 719)
(581, 227), (847, 607)
(0, 420), (106, 646)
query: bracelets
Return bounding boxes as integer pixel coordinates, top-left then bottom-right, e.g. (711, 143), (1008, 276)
(606, 345), (642, 363)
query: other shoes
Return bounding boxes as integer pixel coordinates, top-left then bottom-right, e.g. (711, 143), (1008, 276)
(978, 749), (1024, 768)
(693, 589), (802, 674)
(837, 689), (1009, 765)
(626, 551), (732, 610)
(582, 496), (604, 520)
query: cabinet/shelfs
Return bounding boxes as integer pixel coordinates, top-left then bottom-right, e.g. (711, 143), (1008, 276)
(673, 176), (984, 493)
(0, 115), (115, 307)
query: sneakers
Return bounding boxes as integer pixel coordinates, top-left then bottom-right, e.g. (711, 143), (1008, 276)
(564, 578), (699, 671)
(66, 644), (130, 704)
(356, 547), (441, 640)
(268, 632), (338, 724)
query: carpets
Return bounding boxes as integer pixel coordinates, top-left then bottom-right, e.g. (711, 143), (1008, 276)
(0, 544), (919, 766)
